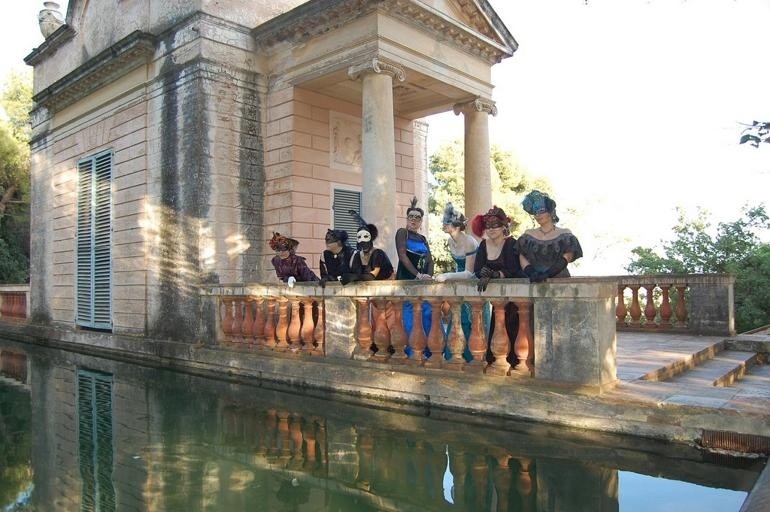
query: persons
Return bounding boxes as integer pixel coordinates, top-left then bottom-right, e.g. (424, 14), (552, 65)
(319, 228), (356, 289)
(435, 202), (490, 363)
(395, 196), (445, 359)
(269, 232), (322, 327)
(513, 190), (583, 284)
(339, 210), (402, 356)
(472, 205), (527, 368)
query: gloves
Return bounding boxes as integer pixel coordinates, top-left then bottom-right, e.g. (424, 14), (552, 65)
(416, 272), (432, 281)
(288, 276), (297, 288)
(524, 256), (568, 282)
(318, 274), (336, 288)
(432, 270), (475, 283)
(477, 267), (499, 292)
(340, 272), (375, 285)
